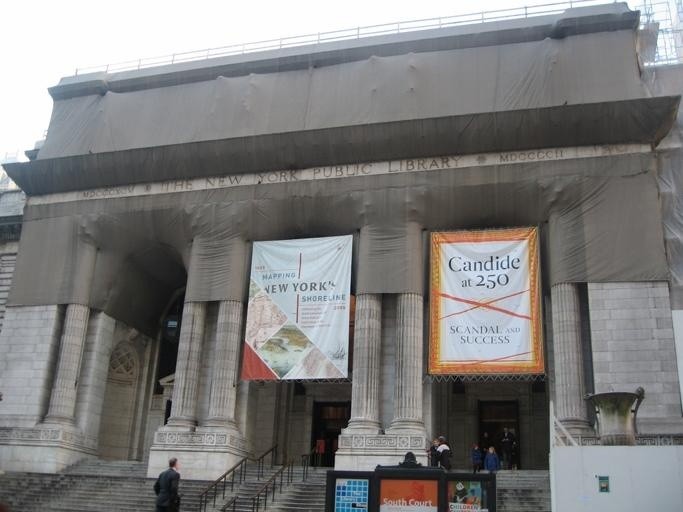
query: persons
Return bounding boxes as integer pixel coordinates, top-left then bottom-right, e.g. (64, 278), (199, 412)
(152, 456), (181, 512)
(430, 424), (514, 473)
